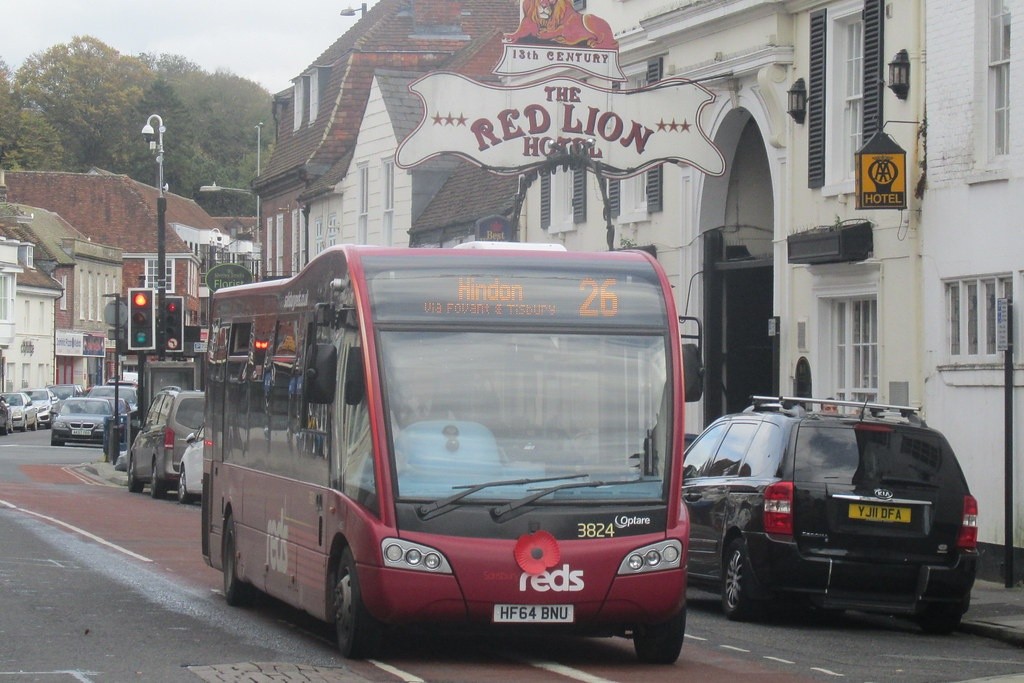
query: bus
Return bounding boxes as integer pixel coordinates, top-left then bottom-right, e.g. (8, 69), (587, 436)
(200, 229), (705, 667)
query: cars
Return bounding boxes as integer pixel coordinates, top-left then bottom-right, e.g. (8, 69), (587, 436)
(1, 376), (136, 454)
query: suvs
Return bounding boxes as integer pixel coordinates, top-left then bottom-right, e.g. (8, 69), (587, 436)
(668, 390), (977, 638)
(128, 384), (207, 495)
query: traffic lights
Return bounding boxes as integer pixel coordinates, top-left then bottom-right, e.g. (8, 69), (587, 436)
(128, 287), (157, 353)
(164, 294), (185, 353)
